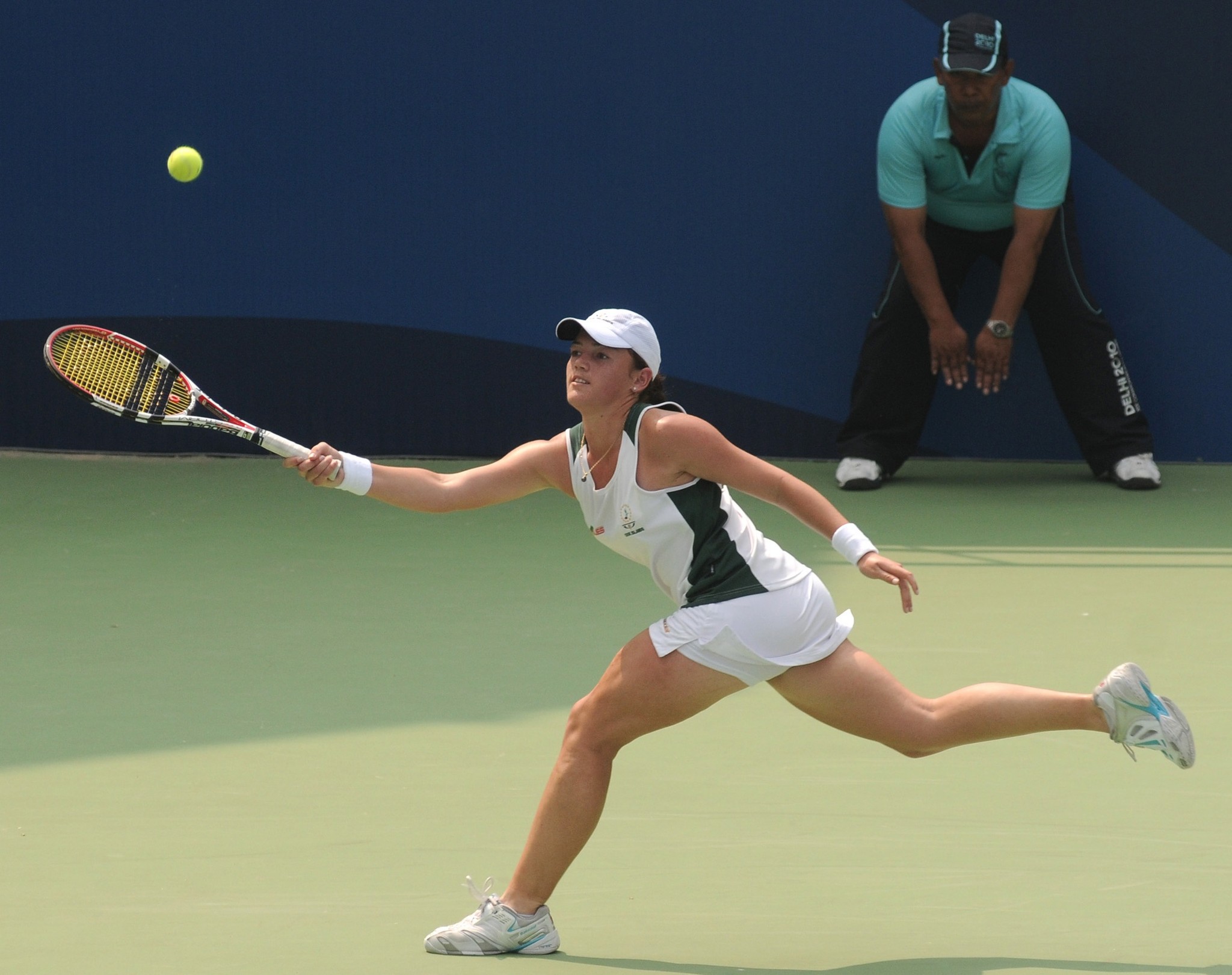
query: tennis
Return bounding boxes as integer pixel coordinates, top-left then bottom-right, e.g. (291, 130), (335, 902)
(167, 146), (204, 183)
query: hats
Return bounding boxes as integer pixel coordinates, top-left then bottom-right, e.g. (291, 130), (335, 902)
(556, 308), (661, 381)
(938, 13), (1008, 77)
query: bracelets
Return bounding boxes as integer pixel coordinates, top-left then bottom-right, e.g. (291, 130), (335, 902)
(831, 522), (878, 567)
(334, 451), (372, 496)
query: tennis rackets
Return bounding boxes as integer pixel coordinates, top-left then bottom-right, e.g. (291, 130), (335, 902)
(43, 323), (341, 484)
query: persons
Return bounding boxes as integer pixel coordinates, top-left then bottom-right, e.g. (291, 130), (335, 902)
(282, 308), (1198, 956)
(837, 14), (1164, 488)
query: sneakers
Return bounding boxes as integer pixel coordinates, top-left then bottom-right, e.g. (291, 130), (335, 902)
(1092, 663), (1195, 769)
(424, 876), (560, 956)
(1100, 452), (1161, 488)
(836, 457), (882, 490)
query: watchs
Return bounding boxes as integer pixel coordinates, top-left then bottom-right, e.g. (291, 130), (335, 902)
(986, 318), (1015, 337)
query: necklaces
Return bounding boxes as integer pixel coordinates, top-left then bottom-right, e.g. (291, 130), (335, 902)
(579, 429), (626, 483)
